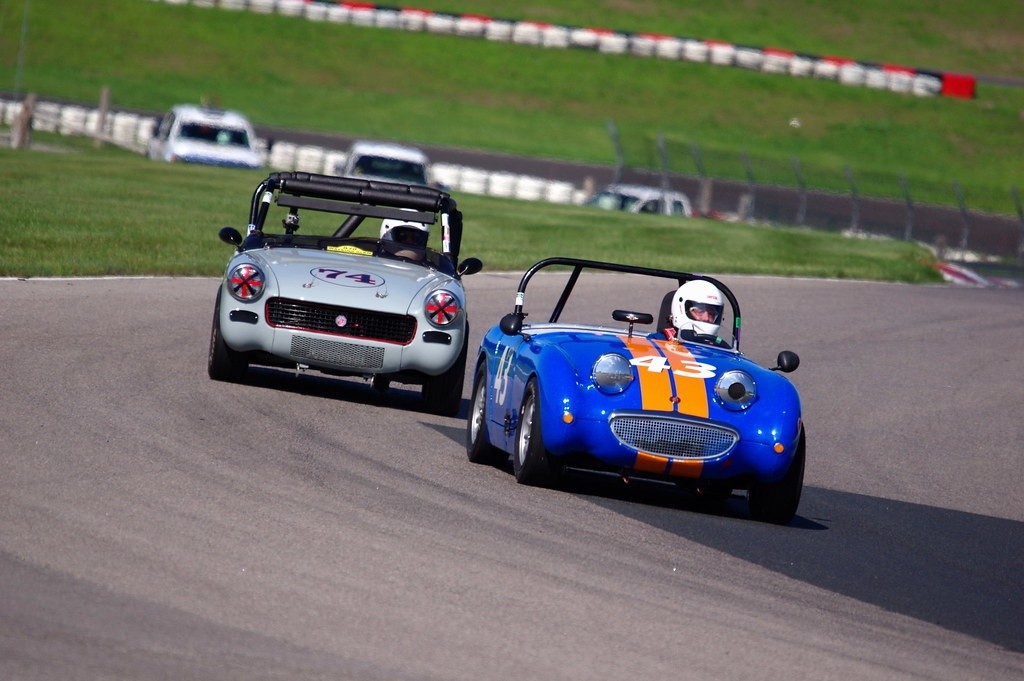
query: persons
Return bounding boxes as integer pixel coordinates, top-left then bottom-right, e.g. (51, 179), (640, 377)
(378, 206), (431, 271)
(645, 280), (732, 352)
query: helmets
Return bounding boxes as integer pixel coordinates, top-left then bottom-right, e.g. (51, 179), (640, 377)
(670, 279), (725, 336)
(378, 205), (430, 247)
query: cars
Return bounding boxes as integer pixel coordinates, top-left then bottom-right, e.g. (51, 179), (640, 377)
(581, 180), (693, 216)
(147, 103), (262, 174)
(206, 173), (483, 416)
(332, 141), (430, 191)
(465, 256), (812, 521)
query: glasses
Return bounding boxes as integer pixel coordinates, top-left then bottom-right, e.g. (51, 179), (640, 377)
(689, 305), (718, 321)
